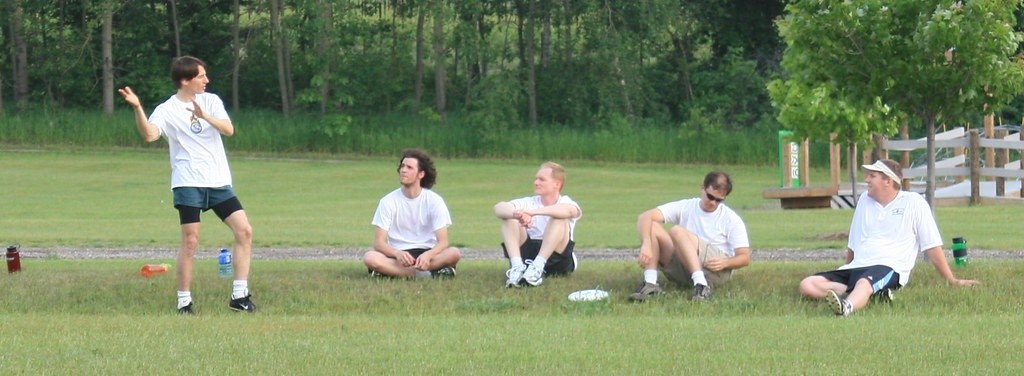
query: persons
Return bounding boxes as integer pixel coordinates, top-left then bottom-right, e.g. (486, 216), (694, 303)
(628, 171), (751, 303)
(799, 160), (978, 318)
(118, 57), (257, 313)
(492, 161), (582, 287)
(364, 149), (462, 282)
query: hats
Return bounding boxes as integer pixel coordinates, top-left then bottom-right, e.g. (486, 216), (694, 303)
(861, 160), (901, 185)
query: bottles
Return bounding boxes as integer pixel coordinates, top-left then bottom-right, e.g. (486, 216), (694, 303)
(952, 237), (969, 266)
(141, 265), (169, 275)
(218, 248), (234, 278)
(6, 246), (21, 272)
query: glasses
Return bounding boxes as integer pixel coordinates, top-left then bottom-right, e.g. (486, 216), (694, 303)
(704, 187), (723, 202)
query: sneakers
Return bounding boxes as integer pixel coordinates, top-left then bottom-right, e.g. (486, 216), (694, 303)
(506, 265), (526, 287)
(228, 293), (256, 313)
(630, 282), (662, 301)
(517, 265), (542, 287)
(825, 289), (846, 320)
(178, 302), (194, 315)
(693, 283), (710, 302)
(430, 266), (456, 279)
(368, 267), (386, 278)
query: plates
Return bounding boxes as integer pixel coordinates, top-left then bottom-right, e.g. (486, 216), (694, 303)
(568, 290), (608, 301)
